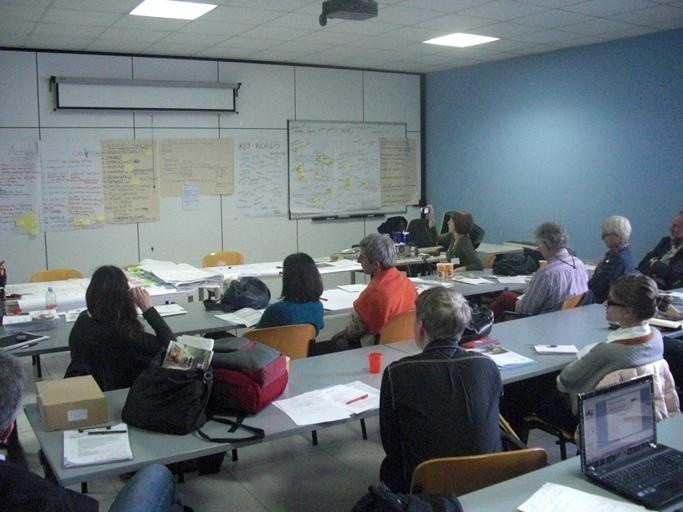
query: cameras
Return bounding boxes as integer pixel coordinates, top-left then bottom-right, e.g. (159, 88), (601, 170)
(418, 206), (432, 216)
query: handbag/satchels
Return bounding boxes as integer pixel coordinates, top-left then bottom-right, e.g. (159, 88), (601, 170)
(122, 346), (266, 444)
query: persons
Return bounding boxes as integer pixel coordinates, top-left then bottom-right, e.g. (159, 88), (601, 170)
(634, 211), (683, 291)
(587, 215), (637, 298)
(557, 272), (670, 448)
(421, 204), (484, 274)
(0, 348), (176, 512)
(252, 252), (325, 339)
(487, 221), (589, 318)
(62, 265), (176, 391)
(354, 286), (504, 512)
(329, 233), (420, 349)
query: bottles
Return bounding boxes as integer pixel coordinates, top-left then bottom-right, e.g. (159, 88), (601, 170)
(45, 287), (57, 310)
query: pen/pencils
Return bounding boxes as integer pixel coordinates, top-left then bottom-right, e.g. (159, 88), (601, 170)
(346, 395), (368, 405)
(320, 297), (327, 301)
(88, 430), (127, 435)
(19, 341), (39, 349)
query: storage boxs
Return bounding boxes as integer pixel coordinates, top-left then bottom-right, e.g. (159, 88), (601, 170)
(35, 374), (108, 433)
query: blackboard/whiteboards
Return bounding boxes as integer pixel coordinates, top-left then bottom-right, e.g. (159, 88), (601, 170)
(286, 116), (410, 221)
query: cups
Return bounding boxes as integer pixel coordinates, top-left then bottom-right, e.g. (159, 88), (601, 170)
(436, 263), (445, 278)
(445, 263), (453, 277)
(368, 352), (382, 374)
(539, 260), (547, 268)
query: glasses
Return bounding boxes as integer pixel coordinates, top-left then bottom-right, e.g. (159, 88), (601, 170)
(608, 295), (625, 308)
(602, 233), (615, 241)
(280, 272), (284, 278)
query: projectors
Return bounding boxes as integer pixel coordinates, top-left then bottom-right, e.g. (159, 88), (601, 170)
(319, 0), (379, 26)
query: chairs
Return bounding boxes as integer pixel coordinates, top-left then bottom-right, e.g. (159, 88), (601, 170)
(524, 359), (681, 460)
(202, 251), (244, 268)
(500, 291), (594, 320)
(368, 431), (549, 512)
(241, 322), (316, 359)
(379, 306), (418, 346)
(28, 268), (82, 284)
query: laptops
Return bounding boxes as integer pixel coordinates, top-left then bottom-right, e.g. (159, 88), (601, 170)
(575, 373), (683, 512)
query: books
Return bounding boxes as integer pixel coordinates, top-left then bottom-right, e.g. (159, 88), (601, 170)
(535, 342), (577, 356)
(161, 341), (213, 372)
(214, 307), (268, 328)
(465, 343), (537, 369)
(136, 302), (187, 318)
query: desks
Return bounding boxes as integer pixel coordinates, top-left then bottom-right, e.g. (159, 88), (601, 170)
(453, 407), (683, 512)
(1, 263), (599, 377)
(0, 241), (532, 382)
(23, 288), (683, 492)
